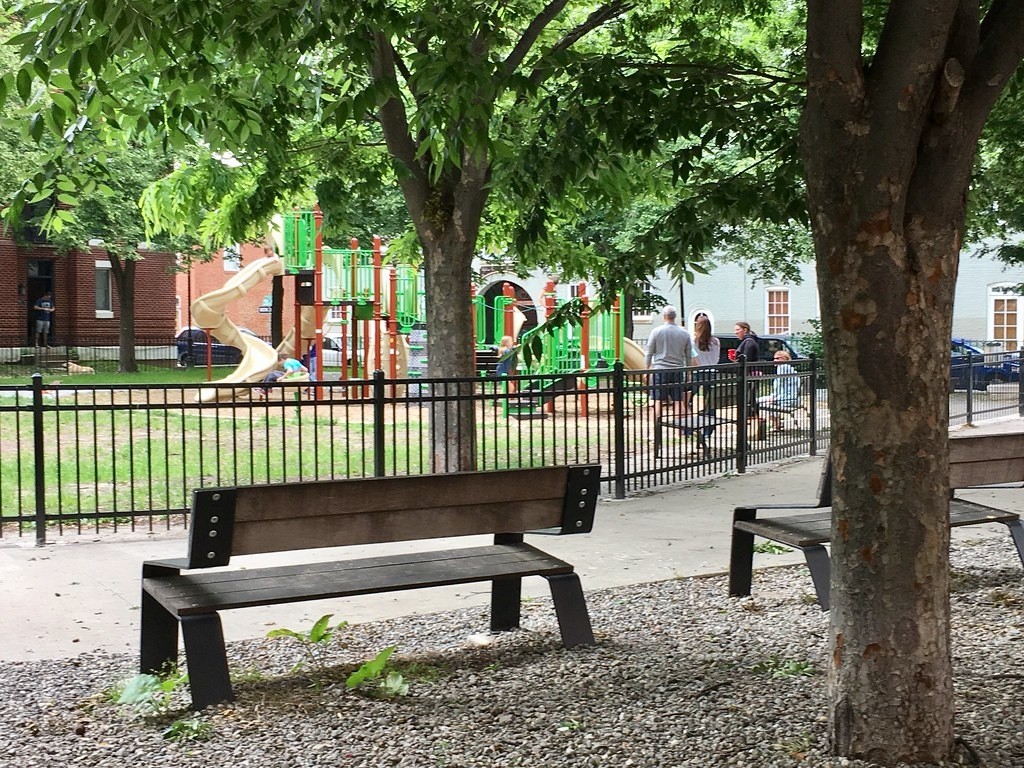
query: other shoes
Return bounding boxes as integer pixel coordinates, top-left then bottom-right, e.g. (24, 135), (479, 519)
(267, 389), (273, 395)
(252, 387), (267, 395)
(767, 426), (784, 432)
(704, 434), (711, 443)
(302, 388), (309, 394)
(310, 392), (315, 396)
(35, 343), (40, 348)
(43, 344), (51, 348)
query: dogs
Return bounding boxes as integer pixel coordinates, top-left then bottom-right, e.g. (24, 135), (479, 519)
(62, 362), (94, 374)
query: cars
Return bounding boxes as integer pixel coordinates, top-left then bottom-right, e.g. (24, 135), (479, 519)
(951, 337), (1022, 391)
(177, 326), (265, 367)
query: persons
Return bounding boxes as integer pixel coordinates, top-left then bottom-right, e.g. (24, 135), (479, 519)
(34, 292), (55, 349)
(734, 322), (760, 425)
(642, 305), (698, 442)
(683, 317), (721, 439)
(756, 350), (801, 435)
(497, 336), (518, 399)
(252, 340), (317, 396)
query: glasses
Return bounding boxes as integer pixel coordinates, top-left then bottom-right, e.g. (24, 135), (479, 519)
(774, 357), (782, 360)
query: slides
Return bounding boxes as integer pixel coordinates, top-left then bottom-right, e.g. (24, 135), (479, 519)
(191, 256), (289, 402)
(622, 336), (663, 394)
(260, 297), (330, 376)
(366, 311), (410, 407)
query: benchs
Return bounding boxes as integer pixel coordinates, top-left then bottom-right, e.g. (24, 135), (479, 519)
(654, 373), (757, 461)
(140, 462), (602, 711)
(759, 377), (814, 436)
(728, 433), (1024, 612)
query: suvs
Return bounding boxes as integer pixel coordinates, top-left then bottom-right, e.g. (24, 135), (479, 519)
(713, 334), (827, 389)
